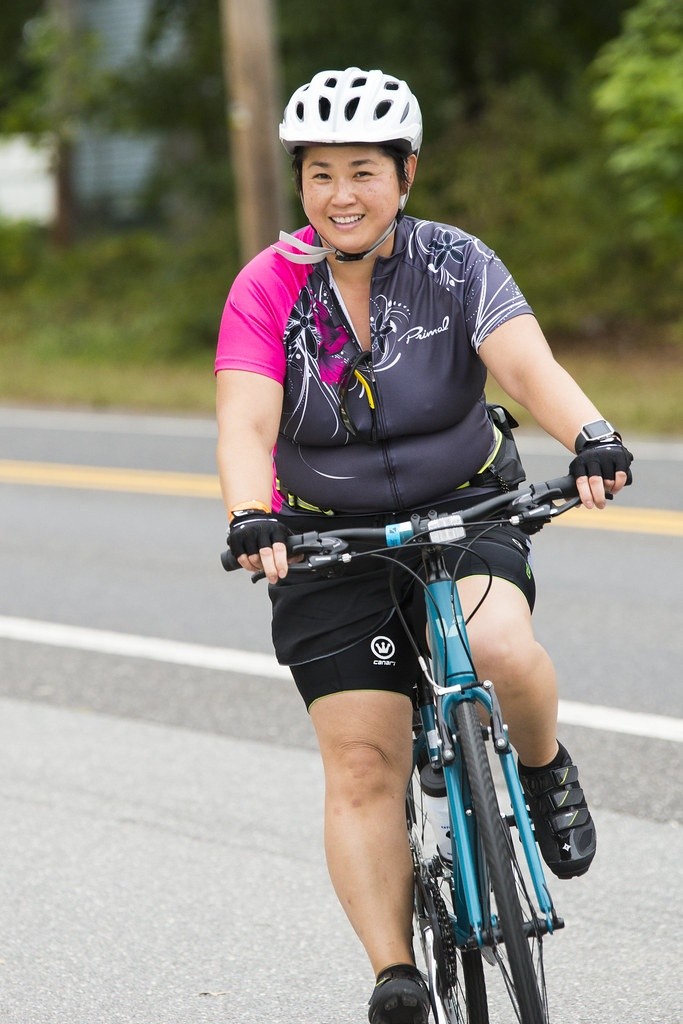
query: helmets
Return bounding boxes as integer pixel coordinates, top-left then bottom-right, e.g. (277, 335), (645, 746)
(278, 67), (423, 157)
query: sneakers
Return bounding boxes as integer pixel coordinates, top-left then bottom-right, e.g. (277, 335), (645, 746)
(518, 739), (598, 880)
(368, 965), (430, 1024)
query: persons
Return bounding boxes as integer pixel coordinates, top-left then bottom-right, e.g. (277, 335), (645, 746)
(213, 67), (627, 1024)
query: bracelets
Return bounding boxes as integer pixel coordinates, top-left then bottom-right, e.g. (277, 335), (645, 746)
(228, 500), (269, 520)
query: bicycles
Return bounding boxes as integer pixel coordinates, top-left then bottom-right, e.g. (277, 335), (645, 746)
(220, 477), (615, 1024)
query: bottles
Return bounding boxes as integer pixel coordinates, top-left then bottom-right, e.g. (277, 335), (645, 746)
(420, 759), (454, 864)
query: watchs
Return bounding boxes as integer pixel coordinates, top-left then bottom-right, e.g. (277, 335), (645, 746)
(574, 420), (623, 455)
